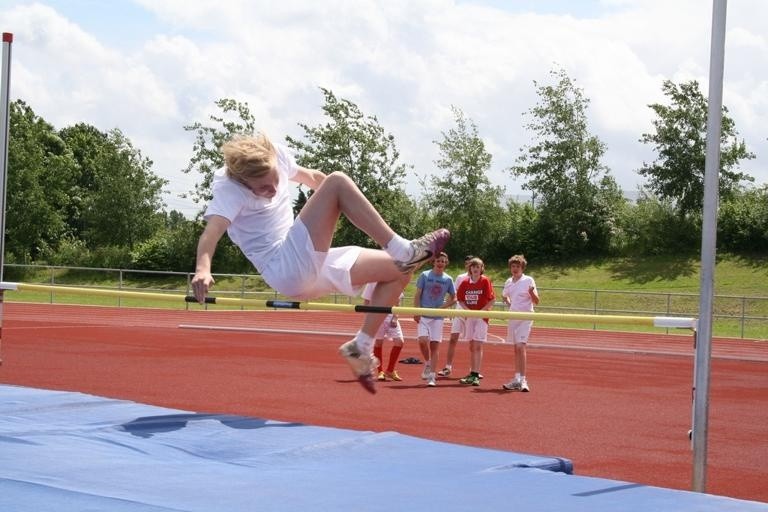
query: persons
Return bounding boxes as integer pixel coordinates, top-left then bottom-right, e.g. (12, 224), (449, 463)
(438, 255), (496, 386)
(191, 131), (451, 394)
(361, 282), (404, 381)
(414, 252), (457, 386)
(501, 254), (539, 392)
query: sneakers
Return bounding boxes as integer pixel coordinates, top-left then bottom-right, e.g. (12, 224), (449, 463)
(422, 365), (436, 387)
(393, 228), (450, 275)
(378, 372), (385, 380)
(503, 379), (530, 391)
(438, 368), (451, 375)
(460, 371), (483, 386)
(385, 369), (402, 381)
(338, 339), (380, 395)
